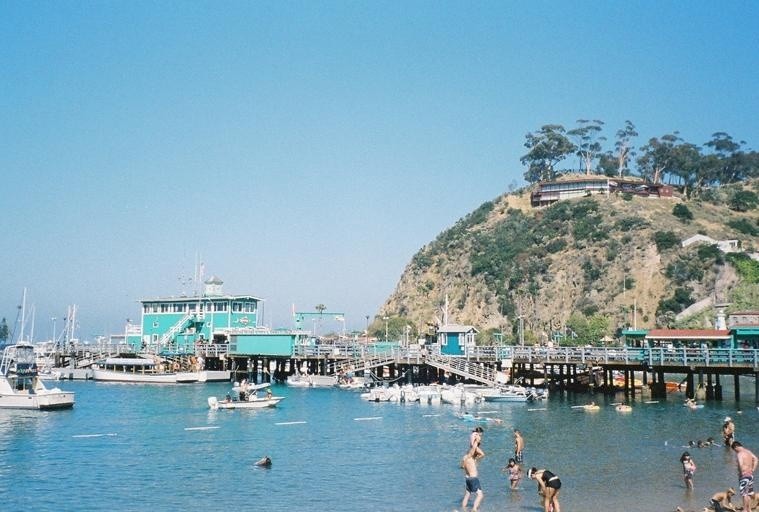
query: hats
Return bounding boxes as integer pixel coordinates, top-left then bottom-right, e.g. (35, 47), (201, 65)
(724, 417), (731, 422)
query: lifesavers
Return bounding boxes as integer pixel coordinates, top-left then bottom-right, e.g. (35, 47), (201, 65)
(616, 405), (631, 412)
(583, 404), (600, 410)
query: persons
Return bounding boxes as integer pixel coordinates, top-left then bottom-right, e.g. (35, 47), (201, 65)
(527, 467), (562, 512)
(534, 335), (754, 415)
(687, 416), (735, 448)
(132, 339), (220, 353)
(707, 441), (759, 512)
(513, 429), (525, 465)
(337, 368), (354, 385)
(470, 427), (485, 465)
(680, 452), (696, 489)
(462, 435), (484, 512)
(240, 379), (246, 401)
(502, 458), (523, 489)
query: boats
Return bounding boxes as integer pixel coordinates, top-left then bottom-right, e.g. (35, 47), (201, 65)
(287, 373), (339, 388)
(358, 383), (549, 406)
(207, 382), (286, 411)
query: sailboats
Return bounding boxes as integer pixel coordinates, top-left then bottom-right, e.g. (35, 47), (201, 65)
(0, 287), (230, 409)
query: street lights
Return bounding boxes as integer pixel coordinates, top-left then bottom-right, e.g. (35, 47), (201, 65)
(312, 318), (316, 335)
(520, 315), (525, 346)
(383, 317), (390, 343)
(365, 315), (369, 346)
